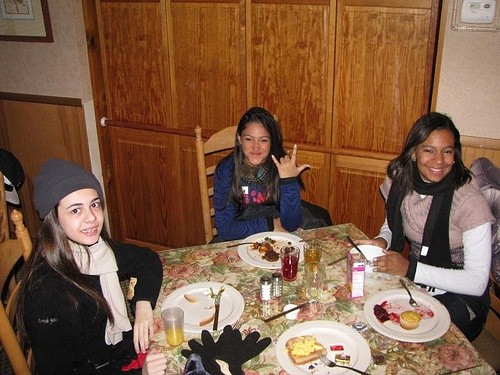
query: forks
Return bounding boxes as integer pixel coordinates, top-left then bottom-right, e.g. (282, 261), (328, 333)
(318, 352), (370, 375)
(398, 278), (420, 308)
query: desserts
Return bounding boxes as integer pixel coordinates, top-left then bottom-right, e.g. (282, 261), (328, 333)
(399, 310), (421, 330)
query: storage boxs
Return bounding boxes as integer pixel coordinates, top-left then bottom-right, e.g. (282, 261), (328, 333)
(347, 250), (366, 298)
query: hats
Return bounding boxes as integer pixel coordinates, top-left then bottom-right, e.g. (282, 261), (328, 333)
(0, 148), (25, 208)
(33, 159), (104, 219)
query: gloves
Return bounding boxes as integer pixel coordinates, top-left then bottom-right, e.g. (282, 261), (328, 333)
(181, 325), (272, 375)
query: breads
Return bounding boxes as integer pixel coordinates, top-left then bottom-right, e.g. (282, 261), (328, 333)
(286, 335), (327, 365)
(264, 249), (280, 261)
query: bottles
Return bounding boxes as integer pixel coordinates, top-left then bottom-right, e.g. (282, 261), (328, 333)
(270, 273), (283, 297)
(258, 276), (272, 302)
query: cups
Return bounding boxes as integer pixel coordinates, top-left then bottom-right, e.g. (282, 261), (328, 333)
(279, 245), (300, 282)
(303, 261), (324, 300)
(161, 307), (185, 347)
(303, 240), (322, 272)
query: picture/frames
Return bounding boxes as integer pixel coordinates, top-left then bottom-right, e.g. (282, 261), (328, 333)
(0, 0), (54, 42)
(450, 0), (500, 32)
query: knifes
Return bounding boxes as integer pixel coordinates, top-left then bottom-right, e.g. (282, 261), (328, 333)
(213, 297), (220, 331)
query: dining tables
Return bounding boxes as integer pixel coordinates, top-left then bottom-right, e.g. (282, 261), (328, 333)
(129, 223), (496, 375)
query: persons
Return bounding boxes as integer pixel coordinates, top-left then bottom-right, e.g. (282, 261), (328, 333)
(212, 106), (311, 240)
(17, 159), (170, 375)
(345, 112), (496, 342)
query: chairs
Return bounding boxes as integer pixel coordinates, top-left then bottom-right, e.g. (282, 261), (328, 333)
(0, 169), (35, 375)
(194, 114), (279, 244)
(470, 157), (500, 370)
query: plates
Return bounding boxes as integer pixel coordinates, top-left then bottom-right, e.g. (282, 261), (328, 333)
(238, 232), (310, 268)
(363, 288), (451, 343)
(160, 281), (245, 334)
(276, 320), (372, 375)
(349, 242), (388, 273)
